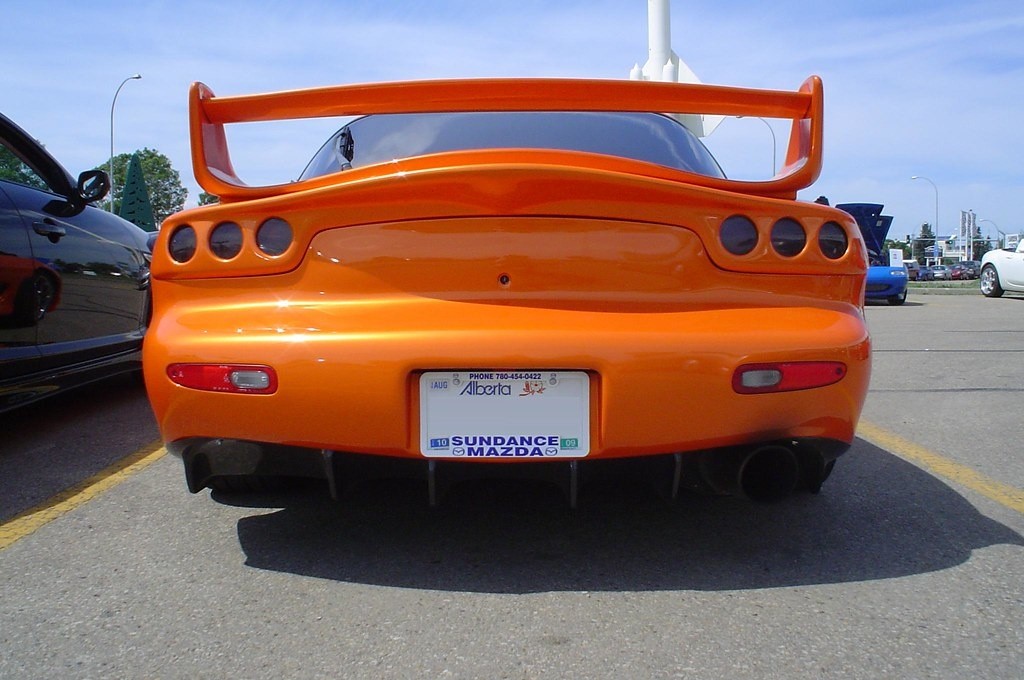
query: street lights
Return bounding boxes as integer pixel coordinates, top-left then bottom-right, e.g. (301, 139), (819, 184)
(979, 219), (1000, 249)
(110, 72), (142, 215)
(912, 175), (938, 266)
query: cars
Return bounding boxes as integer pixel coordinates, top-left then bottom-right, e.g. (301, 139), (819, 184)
(903, 260), (981, 281)
(0, 113), (159, 438)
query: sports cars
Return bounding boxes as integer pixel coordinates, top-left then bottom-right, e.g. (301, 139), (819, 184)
(143, 74), (872, 504)
(978, 239), (1024, 298)
(836, 202), (909, 306)
